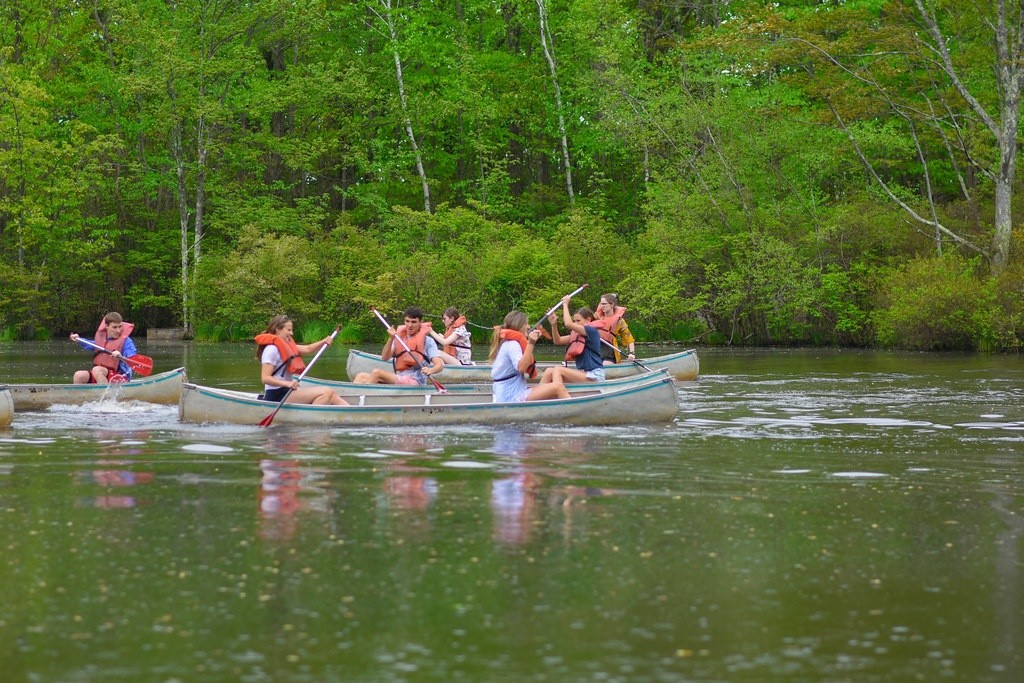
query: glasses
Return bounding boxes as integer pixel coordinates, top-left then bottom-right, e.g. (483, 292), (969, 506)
(442, 317), (450, 321)
(281, 315), (287, 321)
(601, 302), (613, 306)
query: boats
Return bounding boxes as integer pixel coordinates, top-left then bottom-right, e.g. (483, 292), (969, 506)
(0, 388), (15, 428)
(346, 348), (699, 381)
(0, 366), (191, 408)
(290, 365), (677, 394)
(179, 376), (682, 428)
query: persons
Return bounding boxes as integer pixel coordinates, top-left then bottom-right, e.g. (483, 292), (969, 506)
(487, 310), (571, 403)
(352, 307), (444, 385)
(429, 308), (472, 365)
(254, 315), (350, 405)
(70, 311), (137, 384)
(593, 292), (635, 366)
(538, 295), (606, 384)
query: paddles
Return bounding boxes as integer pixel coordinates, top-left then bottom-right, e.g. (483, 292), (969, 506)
(71, 333), (153, 375)
(370, 304), (444, 391)
(256, 325), (342, 429)
(527, 284), (589, 332)
(600, 337), (651, 372)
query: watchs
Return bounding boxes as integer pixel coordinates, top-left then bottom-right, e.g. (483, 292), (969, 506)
(629, 351), (636, 355)
